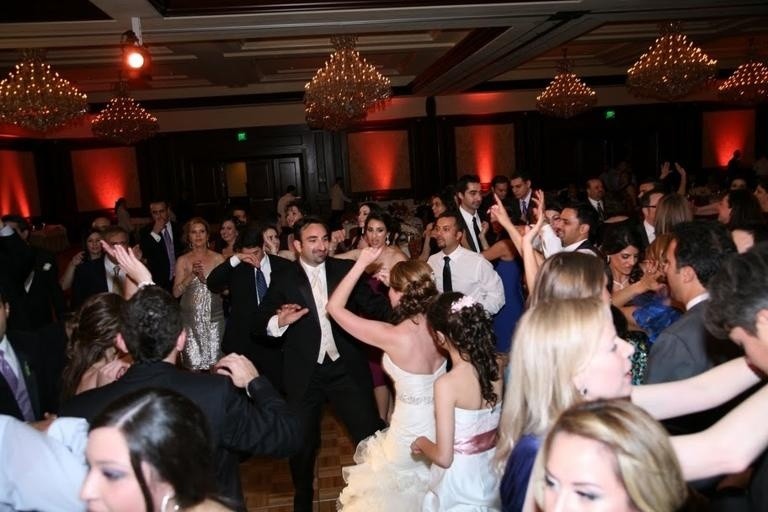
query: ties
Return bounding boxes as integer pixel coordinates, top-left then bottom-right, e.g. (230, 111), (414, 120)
(443, 256), (452, 292)
(309, 269), (335, 351)
(473, 217), (484, 253)
(256, 266), (268, 303)
(112, 265), (123, 298)
(597, 202), (604, 220)
(162, 225), (176, 280)
(522, 201), (527, 215)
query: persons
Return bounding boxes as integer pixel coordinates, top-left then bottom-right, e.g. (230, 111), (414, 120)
(0, 148), (767, 512)
